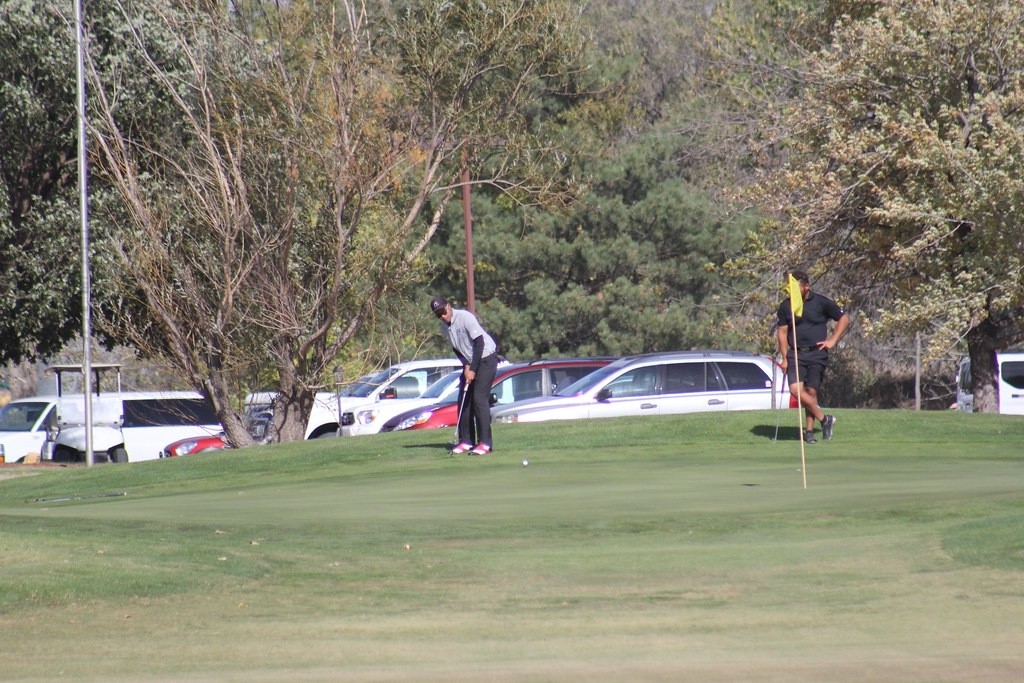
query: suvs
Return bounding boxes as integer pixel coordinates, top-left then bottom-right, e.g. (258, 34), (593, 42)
(490, 350), (803, 424)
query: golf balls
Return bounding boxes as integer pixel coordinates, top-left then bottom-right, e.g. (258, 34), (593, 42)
(523, 460), (528, 465)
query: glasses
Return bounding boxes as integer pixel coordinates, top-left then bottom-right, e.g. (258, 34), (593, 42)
(437, 309), (448, 319)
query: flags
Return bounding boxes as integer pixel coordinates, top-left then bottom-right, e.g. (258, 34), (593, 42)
(790, 276), (804, 317)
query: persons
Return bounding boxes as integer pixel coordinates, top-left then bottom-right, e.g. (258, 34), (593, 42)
(431, 296), (499, 455)
(776, 272), (850, 445)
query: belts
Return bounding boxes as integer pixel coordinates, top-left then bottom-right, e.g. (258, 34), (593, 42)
(791, 345), (820, 352)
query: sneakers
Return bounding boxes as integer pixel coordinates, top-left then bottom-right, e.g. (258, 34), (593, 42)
(468, 441), (492, 456)
(803, 429), (818, 444)
(448, 442), (476, 455)
(821, 414), (834, 440)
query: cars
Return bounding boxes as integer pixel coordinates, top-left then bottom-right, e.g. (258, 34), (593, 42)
(164, 359), (617, 456)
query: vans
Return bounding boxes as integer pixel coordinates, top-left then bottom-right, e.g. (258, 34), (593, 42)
(957, 353), (1024, 414)
(0, 392), (224, 462)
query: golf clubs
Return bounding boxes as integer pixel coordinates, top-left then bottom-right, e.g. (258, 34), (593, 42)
(772, 374), (786, 443)
(447, 380), (469, 455)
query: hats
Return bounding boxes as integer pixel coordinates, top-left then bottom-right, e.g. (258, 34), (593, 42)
(430, 297), (447, 314)
(783, 271), (809, 283)
